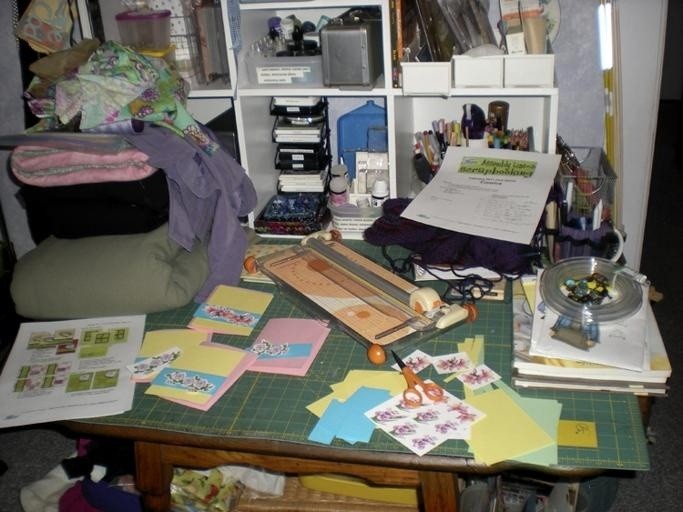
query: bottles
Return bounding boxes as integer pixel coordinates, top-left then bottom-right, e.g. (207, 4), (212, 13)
(489, 101), (509, 131)
(249, 14), (331, 58)
(329, 165), (390, 210)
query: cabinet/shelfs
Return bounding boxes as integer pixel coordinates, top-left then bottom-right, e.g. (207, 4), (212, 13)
(11, 0), (626, 250)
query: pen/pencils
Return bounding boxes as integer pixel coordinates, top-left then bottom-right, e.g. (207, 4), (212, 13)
(414, 119), (529, 176)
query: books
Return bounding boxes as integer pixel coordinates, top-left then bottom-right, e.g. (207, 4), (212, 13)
(270, 97), (330, 193)
(510, 267), (673, 399)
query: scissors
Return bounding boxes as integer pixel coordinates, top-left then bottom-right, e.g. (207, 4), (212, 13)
(391, 351), (442, 407)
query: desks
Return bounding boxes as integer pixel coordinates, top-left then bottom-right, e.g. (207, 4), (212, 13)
(25, 238), (651, 512)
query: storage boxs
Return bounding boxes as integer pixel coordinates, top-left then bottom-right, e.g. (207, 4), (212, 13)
(319, 6), (383, 86)
(451, 54), (504, 89)
(504, 53), (556, 88)
(400, 47), (452, 97)
